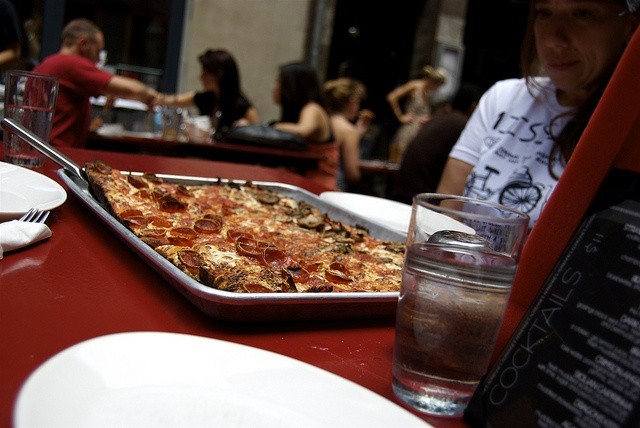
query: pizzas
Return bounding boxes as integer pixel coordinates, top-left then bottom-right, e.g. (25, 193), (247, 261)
(83, 159), (407, 294)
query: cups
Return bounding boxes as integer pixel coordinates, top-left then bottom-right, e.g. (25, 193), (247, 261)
(394, 193), (531, 416)
(5, 69), (58, 175)
(162, 106), (182, 141)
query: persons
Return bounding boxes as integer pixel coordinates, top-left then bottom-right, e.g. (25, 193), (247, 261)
(269, 60), (337, 191)
(392, 87), (480, 205)
(20, 19), (161, 151)
(435, 0), (640, 246)
(387, 65), (446, 163)
(322, 77), (369, 191)
(2, 1), (43, 69)
(160, 48), (259, 144)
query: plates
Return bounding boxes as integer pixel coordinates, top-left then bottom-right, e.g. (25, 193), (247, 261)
(12, 332), (439, 427)
(321, 190), (475, 241)
(1, 161), (69, 214)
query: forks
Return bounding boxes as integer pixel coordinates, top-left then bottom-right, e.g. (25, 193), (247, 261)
(19, 207), (53, 226)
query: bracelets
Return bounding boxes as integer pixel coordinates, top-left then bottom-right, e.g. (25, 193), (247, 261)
(270, 120), (278, 128)
(26, 32), (37, 40)
